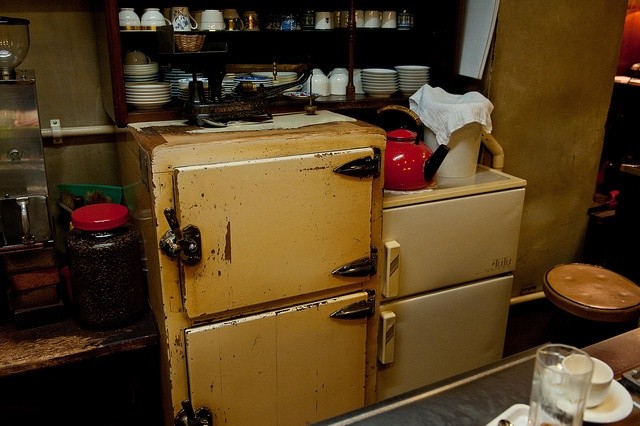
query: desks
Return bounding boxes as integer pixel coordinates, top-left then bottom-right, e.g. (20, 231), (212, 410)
(308, 328), (638, 426)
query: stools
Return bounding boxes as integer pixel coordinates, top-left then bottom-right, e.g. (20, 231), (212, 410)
(541, 262), (640, 350)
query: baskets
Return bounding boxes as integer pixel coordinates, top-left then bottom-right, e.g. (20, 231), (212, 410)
(175, 33), (205, 54)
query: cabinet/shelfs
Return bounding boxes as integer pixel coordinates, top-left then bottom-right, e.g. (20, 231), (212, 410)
(91, 0), (469, 129)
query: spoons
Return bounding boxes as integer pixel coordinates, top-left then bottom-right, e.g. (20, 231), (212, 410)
(497, 418), (513, 425)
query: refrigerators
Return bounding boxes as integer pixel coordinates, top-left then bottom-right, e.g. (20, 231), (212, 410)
(375, 161), (527, 405)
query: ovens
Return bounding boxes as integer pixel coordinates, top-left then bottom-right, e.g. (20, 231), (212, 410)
(115, 107), (387, 425)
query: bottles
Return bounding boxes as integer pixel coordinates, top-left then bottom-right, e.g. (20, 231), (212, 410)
(279, 14), (297, 31)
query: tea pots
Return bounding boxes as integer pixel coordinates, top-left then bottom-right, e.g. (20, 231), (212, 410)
(373, 104), (450, 190)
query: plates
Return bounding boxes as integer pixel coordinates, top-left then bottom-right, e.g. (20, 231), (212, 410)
(228, 66), (296, 95)
(282, 90), (323, 99)
(123, 61), (172, 108)
(542, 365), (634, 423)
(395, 64), (431, 95)
(160, 64), (236, 104)
(361, 68), (399, 97)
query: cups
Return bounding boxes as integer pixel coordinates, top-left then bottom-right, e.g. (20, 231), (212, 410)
(340, 10), (349, 28)
(556, 354), (614, 408)
(301, 9), (314, 31)
(119, 8), (141, 32)
(307, 67), (364, 94)
(364, 10), (382, 28)
(223, 8), (244, 31)
(169, 6), (198, 32)
(162, 7), (171, 23)
(124, 50), (151, 63)
(198, 9), (226, 31)
(333, 11), (341, 27)
(140, 7), (172, 32)
(193, 10), (202, 30)
(242, 10), (260, 32)
(527, 342), (594, 425)
(398, 10), (414, 30)
(314, 11), (332, 29)
(381, 10), (397, 28)
(353, 9), (364, 28)
